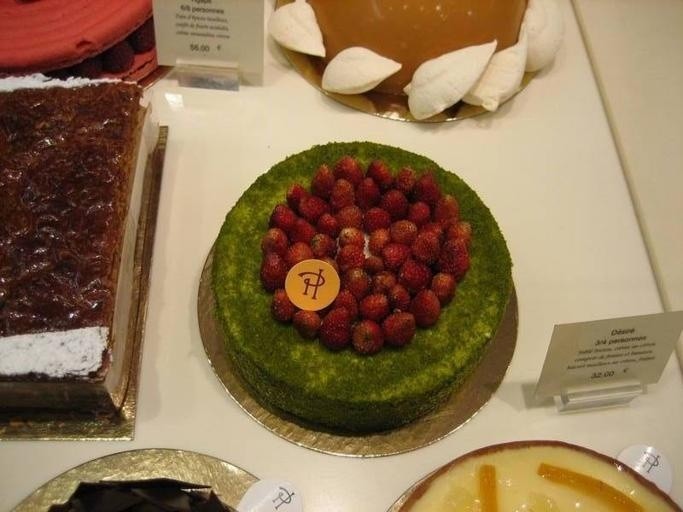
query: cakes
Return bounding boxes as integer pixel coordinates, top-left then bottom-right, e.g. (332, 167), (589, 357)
(1, 1), (174, 94)
(268, 1), (567, 123)
(0, 72), (170, 440)
(387, 439), (683, 511)
(209, 140), (512, 437)
